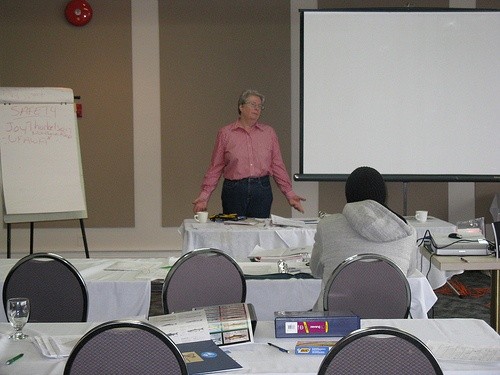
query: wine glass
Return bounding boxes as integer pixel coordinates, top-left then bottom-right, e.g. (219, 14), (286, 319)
(7, 298), (30, 340)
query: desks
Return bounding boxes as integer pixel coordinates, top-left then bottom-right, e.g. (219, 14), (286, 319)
(0, 258), (438, 324)
(0, 317), (500, 375)
(420, 245), (500, 334)
(180, 216), (457, 257)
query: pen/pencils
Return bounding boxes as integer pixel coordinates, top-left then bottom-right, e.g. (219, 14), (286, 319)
(461, 257), (469, 263)
(267, 342), (290, 353)
(5, 352), (24, 366)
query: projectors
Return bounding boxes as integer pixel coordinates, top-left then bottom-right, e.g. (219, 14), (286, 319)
(426, 226), (489, 256)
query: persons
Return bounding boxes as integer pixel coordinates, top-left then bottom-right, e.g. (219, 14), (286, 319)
(309, 166), (417, 313)
(192, 89), (307, 218)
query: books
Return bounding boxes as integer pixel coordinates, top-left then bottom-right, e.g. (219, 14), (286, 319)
(175, 340), (243, 375)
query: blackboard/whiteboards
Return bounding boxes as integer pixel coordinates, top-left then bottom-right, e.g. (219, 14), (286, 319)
(0, 85), (88, 226)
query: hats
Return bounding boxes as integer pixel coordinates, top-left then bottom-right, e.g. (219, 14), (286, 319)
(345, 166), (386, 202)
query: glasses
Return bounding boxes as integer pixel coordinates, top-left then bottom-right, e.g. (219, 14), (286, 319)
(244, 101), (264, 110)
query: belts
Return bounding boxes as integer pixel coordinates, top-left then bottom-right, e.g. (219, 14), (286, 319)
(225, 177), (259, 184)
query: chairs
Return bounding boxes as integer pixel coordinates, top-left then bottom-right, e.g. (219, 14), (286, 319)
(317, 325), (444, 375)
(63, 320), (190, 375)
(162, 248), (248, 313)
(2, 252), (89, 323)
(323, 253), (412, 319)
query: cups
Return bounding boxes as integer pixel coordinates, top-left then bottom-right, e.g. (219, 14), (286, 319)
(415, 211), (428, 223)
(194, 212), (209, 224)
